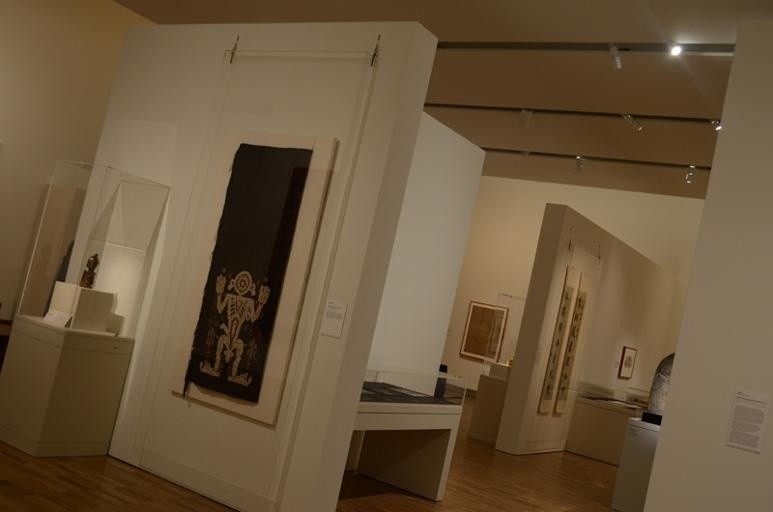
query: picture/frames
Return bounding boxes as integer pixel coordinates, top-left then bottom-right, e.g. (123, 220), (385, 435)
(459, 301), (509, 364)
(618, 346), (638, 379)
